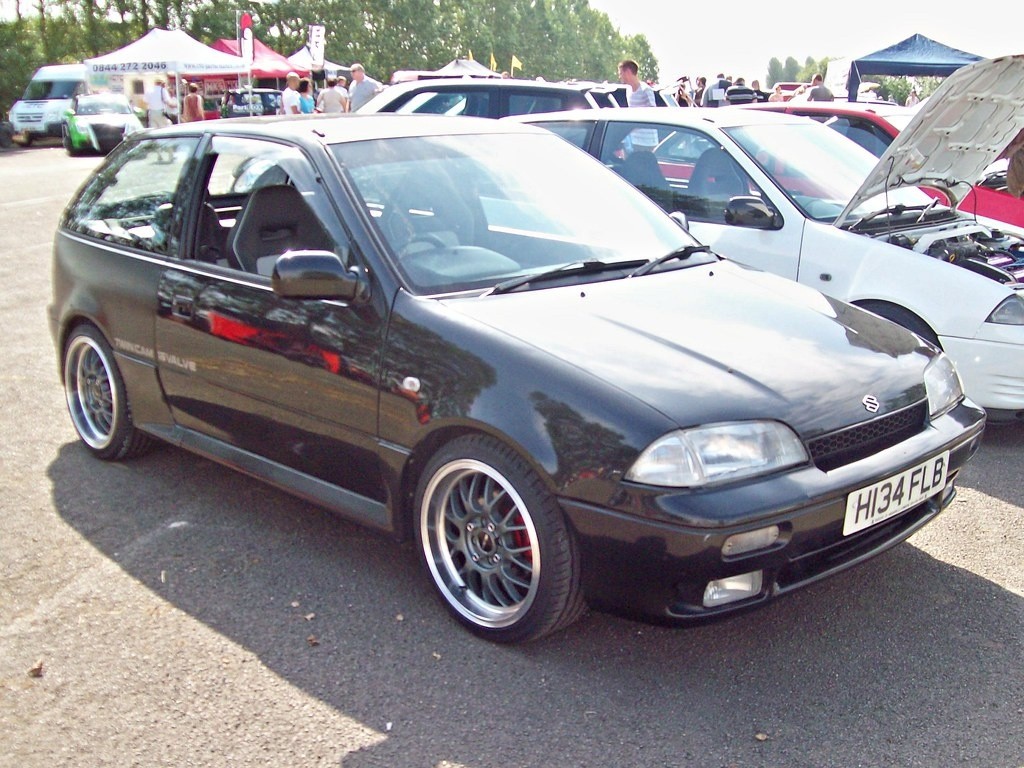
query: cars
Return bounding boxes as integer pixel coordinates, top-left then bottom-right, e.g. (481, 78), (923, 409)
(654, 95), (1024, 231)
(426, 54), (1024, 430)
(59, 91), (146, 158)
(219, 86), (285, 119)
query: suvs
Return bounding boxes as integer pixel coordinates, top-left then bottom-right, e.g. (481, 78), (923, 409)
(47, 112), (988, 645)
(230, 76), (635, 213)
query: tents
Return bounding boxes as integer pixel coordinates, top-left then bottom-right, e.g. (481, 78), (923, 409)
(204, 37), (313, 92)
(84, 27), (250, 125)
(390, 60), (502, 84)
(848, 34), (988, 104)
(287, 47), (352, 85)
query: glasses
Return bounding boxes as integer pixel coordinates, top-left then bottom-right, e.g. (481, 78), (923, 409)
(351, 70), (357, 72)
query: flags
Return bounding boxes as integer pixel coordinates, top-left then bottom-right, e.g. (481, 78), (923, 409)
(491, 53), (498, 71)
(512, 55), (522, 70)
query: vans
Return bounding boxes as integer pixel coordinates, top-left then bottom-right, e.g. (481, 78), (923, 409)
(8, 62), (169, 150)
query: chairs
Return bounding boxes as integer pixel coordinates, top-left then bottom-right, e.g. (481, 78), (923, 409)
(152, 202), (229, 268)
(379, 165), (475, 259)
(617, 150), (673, 214)
(225, 185), (334, 277)
(688, 148), (743, 220)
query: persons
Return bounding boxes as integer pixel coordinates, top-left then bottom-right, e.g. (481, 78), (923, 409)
(143, 62), (382, 129)
(618, 58), (919, 107)
